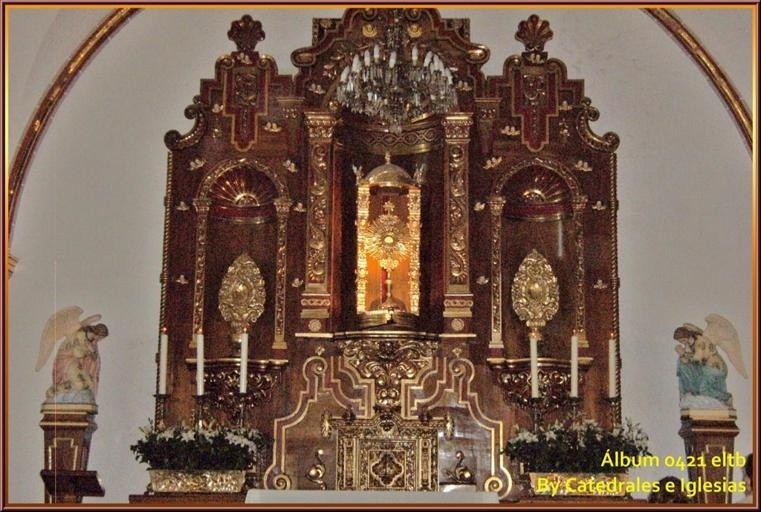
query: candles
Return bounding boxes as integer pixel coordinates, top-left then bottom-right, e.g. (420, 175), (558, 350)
(571, 328), (580, 399)
(239, 327), (249, 393)
(607, 333), (619, 400)
(195, 327), (204, 396)
(158, 327), (168, 396)
(529, 328), (539, 399)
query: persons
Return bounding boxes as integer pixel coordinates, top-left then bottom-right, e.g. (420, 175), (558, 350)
(46, 324), (108, 405)
(673, 327), (733, 408)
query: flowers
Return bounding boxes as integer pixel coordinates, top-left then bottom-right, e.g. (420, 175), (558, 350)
(500, 409), (656, 472)
(129, 414), (274, 470)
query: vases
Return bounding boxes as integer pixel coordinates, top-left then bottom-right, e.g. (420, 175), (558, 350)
(528, 472), (629, 499)
(146, 468), (246, 494)
